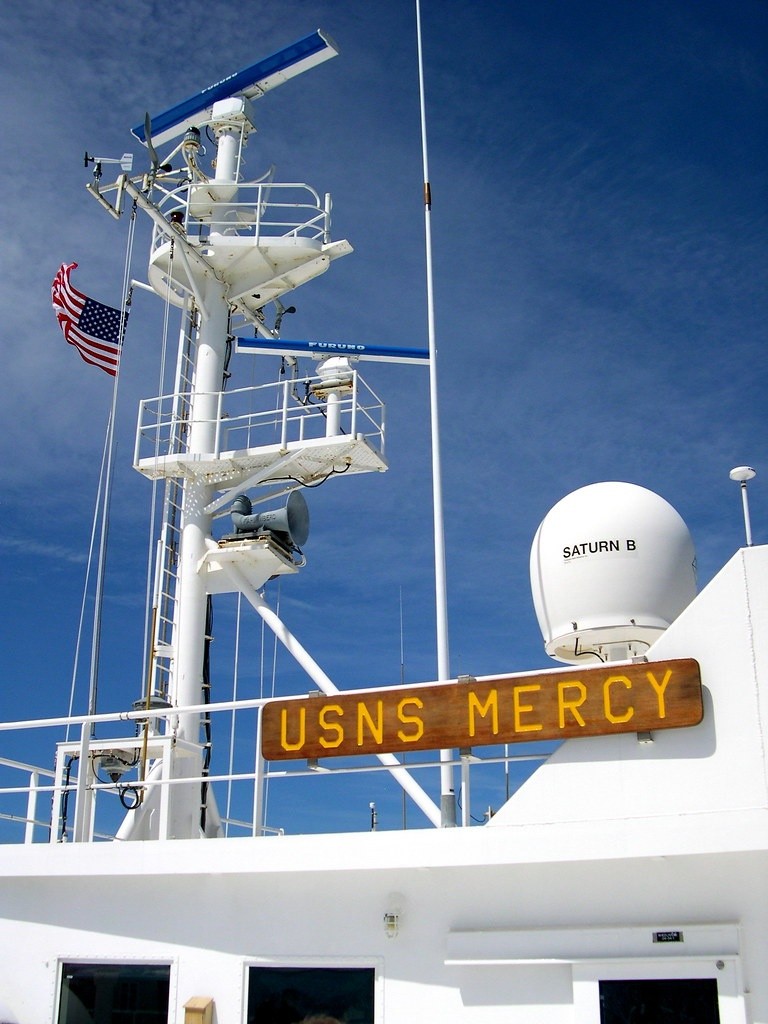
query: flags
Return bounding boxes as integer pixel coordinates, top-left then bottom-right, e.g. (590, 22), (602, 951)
(51, 261), (129, 378)
